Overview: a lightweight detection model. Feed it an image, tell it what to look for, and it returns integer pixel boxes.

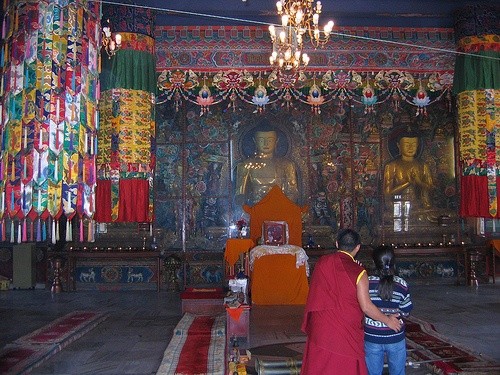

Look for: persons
[234,120,298,201]
[301,229,403,375]
[362,246,413,375]
[383,125,436,224]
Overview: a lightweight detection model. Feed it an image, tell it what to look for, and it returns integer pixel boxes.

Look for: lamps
[102,19,121,59]
[267,0,334,71]
